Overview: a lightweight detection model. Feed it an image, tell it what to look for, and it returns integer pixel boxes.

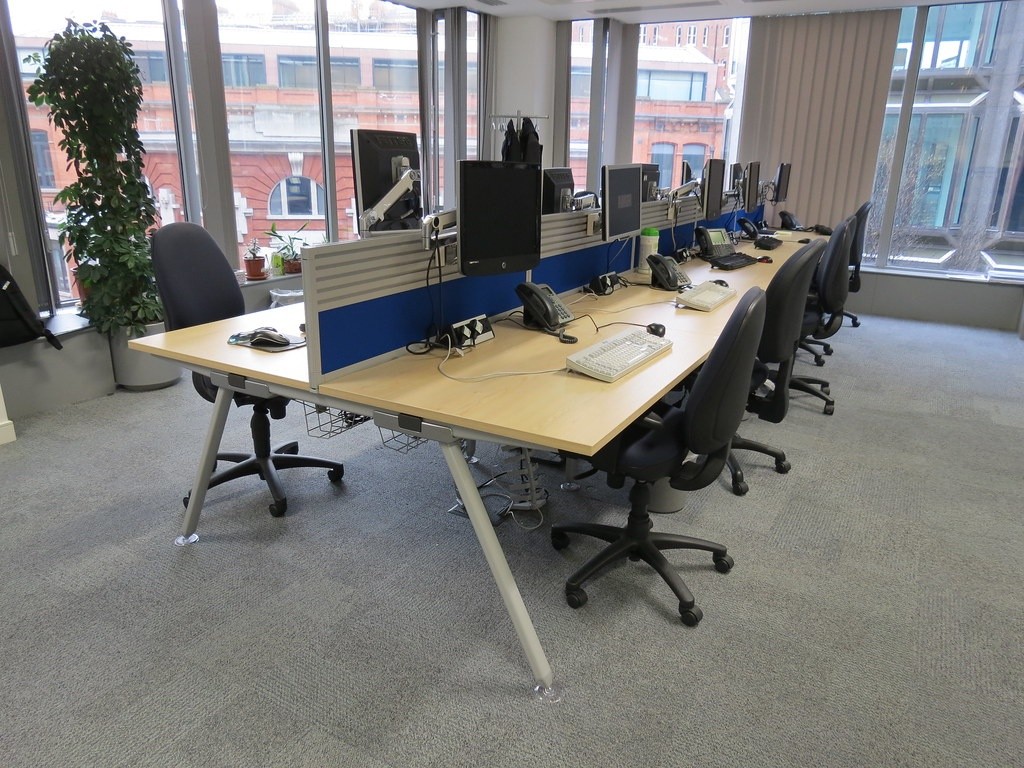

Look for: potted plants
[22,17,181,391]
[243,237,266,279]
[266,221,310,274]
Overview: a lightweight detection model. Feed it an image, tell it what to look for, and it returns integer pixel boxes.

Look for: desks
[127,191,835,704]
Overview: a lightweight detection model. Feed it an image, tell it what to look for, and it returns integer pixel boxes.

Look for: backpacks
[0,264,63,350]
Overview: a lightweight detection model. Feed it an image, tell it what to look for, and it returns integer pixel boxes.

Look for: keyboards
[710,252,758,270]
[675,281,736,312]
[754,236,783,250]
[566,327,673,383]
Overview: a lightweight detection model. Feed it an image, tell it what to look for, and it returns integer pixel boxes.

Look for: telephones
[694,226,735,257]
[645,253,693,290]
[779,210,800,228]
[515,282,575,330]
[736,217,759,238]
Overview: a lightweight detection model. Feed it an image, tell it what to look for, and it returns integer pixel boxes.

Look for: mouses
[250,329,290,346]
[646,323,665,337]
[713,279,728,287]
[798,239,812,243]
[757,255,773,263]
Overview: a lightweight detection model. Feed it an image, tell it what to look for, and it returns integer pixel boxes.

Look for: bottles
[639,228,660,274]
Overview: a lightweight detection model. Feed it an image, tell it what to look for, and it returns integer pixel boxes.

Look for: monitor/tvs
[681,160,691,196]
[541,167,575,215]
[773,163,792,202]
[703,159,725,221]
[350,129,423,236]
[642,163,660,202]
[601,163,641,241]
[729,162,743,191]
[742,161,760,213]
[455,159,541,276]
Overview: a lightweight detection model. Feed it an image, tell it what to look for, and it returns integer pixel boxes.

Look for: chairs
[757,214,858,415]
[843,201,874,329]
[548,286,766,628]
[667,237,829,496]
[149,221,345,522]
